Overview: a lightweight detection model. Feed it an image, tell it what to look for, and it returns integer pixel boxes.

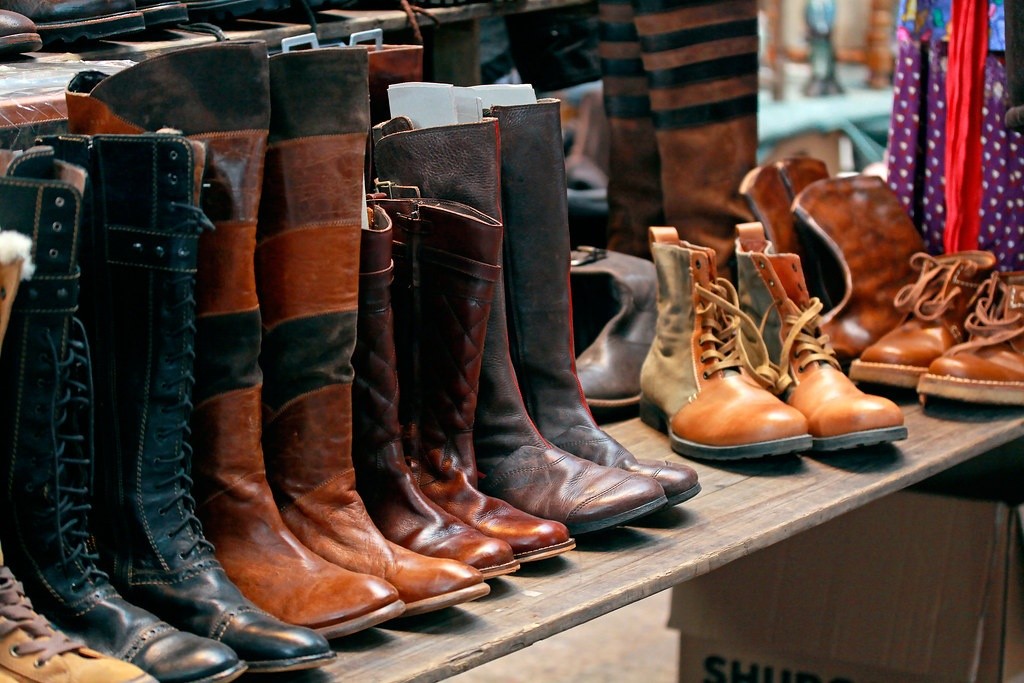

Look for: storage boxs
[666,489,1024,683]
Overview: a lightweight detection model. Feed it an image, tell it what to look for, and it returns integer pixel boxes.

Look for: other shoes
[0,0,290,55]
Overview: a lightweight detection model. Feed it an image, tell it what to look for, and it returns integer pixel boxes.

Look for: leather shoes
[849,250,999,389]
[916,270,1024,405]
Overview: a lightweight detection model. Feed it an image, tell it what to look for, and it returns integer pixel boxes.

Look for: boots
[0,14,701,683]
[571,0,930,455]
